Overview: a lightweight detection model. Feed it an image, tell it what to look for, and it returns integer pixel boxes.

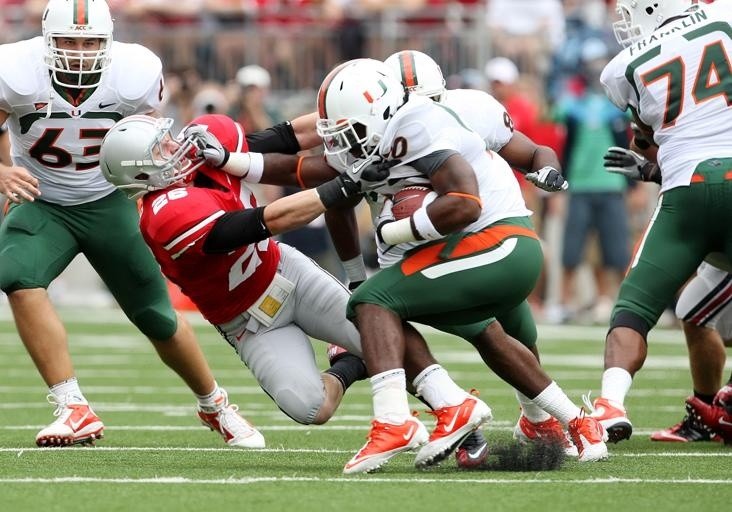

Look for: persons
[2,0,661,324]
[100,51,611,473]
[2,0,269,451]
[582,0,732,451]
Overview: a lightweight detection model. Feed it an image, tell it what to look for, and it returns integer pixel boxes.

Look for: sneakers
[416,393,491,469]
[344,416,429,474]
[199,406,268,448]
[514,412,578,460]
[687,388,729,443]
[35,405,104,447]
[456,431,488,467]
[567,415,608,463]
[652,408,721,442]
[592,399,634,443]
[535,301,613,324]
[327,342,372,380]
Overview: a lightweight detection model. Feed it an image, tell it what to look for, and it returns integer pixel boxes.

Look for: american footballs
[391,186,440,220]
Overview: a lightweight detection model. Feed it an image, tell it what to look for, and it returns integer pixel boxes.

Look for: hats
[486,57,521,83]
[238,66,271,87]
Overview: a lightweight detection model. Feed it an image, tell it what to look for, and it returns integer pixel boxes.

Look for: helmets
[314,56,404,180]
[611,2,689,48]
[99,115,199,197]
[40,0,116,91]
[385,50,447,99]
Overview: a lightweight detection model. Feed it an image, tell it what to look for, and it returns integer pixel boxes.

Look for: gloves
[374,200,395,238]
[525,166,569,193]
[339,156,401,199]
[603,146,656,181]
[190,126,227,169]
